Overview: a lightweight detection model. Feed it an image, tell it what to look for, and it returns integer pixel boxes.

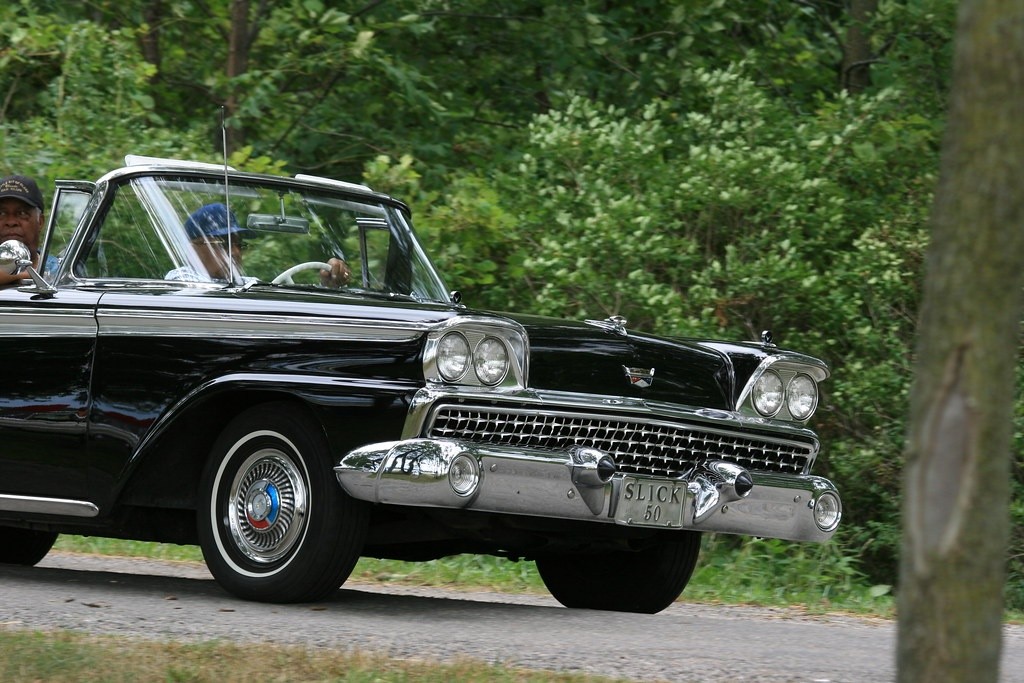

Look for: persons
[164,202,352,289]
[0,176,59,290]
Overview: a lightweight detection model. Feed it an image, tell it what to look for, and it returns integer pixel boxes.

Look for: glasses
[193,237,249,252]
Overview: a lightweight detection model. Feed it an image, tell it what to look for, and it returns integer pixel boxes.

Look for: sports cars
[0,154,844,614]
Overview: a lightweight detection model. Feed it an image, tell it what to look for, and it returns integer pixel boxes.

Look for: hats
[0,174,44,213]
[185,203,251,240]
